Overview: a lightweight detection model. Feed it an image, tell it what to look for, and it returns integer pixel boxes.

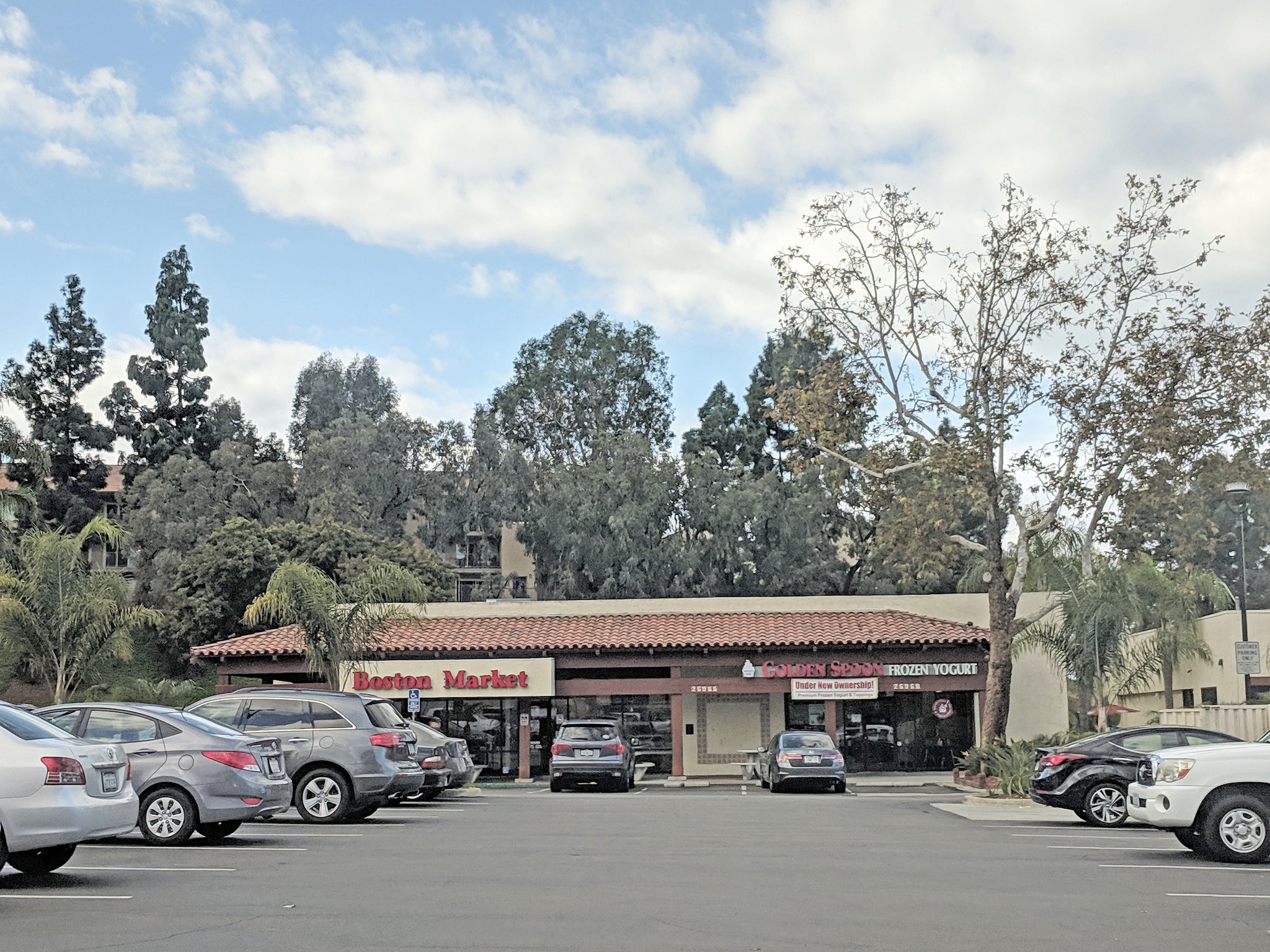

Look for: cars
[401,718,474,801]
[1030,725,1247,827]
[0,697,294,871]
[757,729,847,794]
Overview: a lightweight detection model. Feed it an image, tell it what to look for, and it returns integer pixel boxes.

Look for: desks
[730,748,760,795]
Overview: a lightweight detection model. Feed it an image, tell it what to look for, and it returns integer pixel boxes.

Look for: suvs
[1127,724,1269,865]
[426,707,501,744]
[549,719,635,792]
[171,686,425,824]
